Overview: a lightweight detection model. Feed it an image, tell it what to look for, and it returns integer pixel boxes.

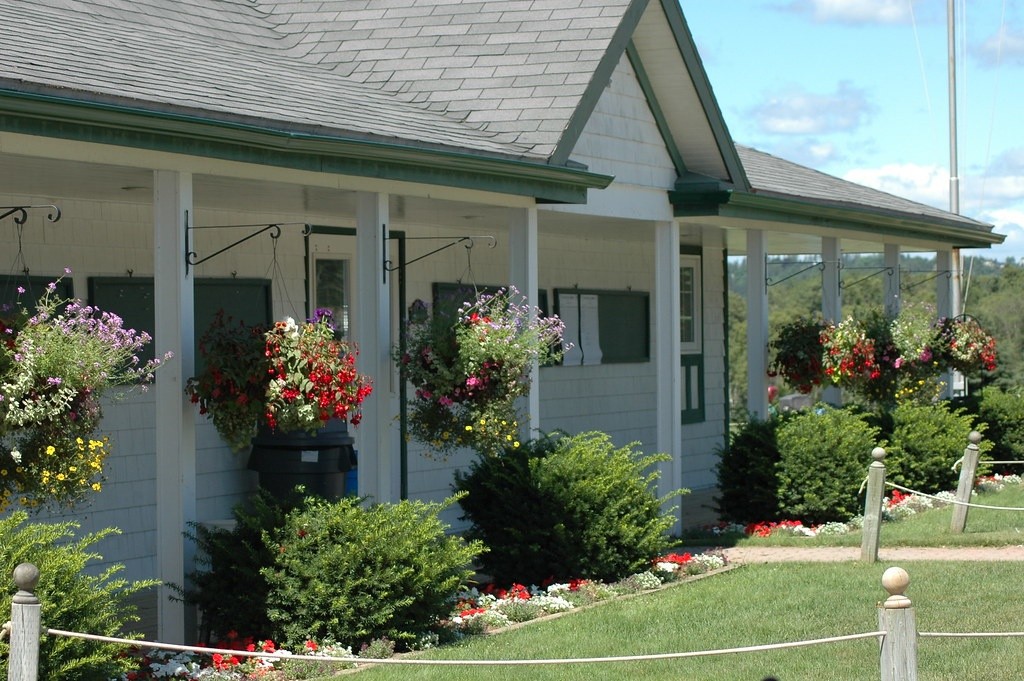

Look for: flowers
[763,294,998,407]
[389,285,576,466]
[181,306,372,456]
[0,265,178,524]
